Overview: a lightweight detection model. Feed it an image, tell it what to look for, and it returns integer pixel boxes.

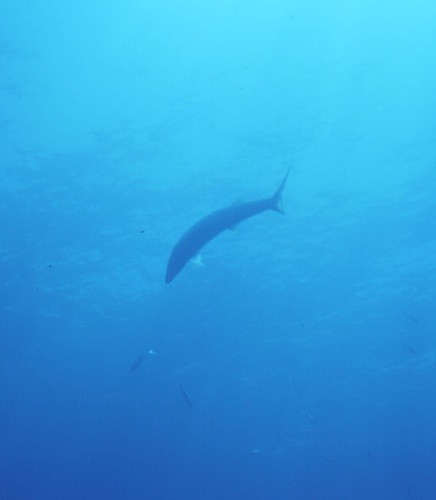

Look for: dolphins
[178,384,194,410]
[165,166,291,286]
[129,350,183,373]
